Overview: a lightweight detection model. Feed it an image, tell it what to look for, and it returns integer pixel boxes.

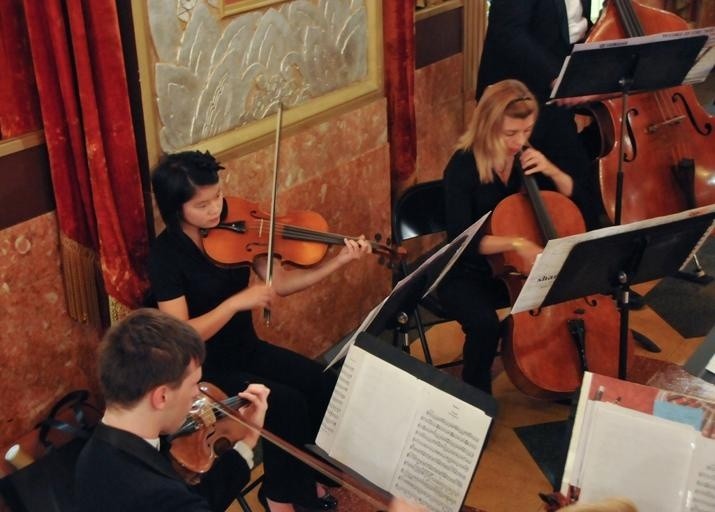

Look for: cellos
[487,149,635,399]
[582,1,715,225]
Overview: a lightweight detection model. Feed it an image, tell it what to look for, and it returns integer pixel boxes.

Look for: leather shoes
[318,489,339,511]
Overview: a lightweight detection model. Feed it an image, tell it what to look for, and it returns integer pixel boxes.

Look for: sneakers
[628,288,646,310]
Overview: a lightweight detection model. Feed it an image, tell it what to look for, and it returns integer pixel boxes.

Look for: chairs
[136,289,265,512]
[392,180,504,371]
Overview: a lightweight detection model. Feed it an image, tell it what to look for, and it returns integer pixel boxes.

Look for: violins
[168,381,249,485]
[201,196,408,269]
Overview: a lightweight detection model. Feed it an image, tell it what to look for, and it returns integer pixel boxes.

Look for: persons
[72,309,270,512]
[147,152,371,512]
[476,0,643,310]
[442,80,587,397]
